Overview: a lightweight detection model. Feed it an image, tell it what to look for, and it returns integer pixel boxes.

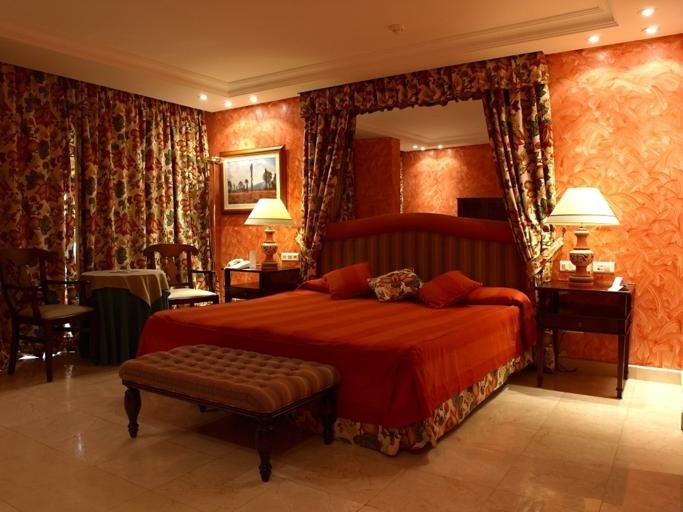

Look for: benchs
[119,344,341,482]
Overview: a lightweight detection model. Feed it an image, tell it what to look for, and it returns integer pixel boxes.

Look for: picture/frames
[219,145,286,215]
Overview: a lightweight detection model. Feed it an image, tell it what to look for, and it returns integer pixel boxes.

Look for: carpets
[204,412,316,458]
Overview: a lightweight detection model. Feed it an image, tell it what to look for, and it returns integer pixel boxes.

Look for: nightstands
[534,280,636,398]
[221,266,300,303]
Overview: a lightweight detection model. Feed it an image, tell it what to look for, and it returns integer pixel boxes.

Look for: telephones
[223,259,251,270]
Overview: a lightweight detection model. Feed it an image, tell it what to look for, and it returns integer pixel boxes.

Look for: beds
[135,212,537,457]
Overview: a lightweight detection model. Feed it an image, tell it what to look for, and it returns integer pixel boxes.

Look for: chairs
[0,246,96,382]
[144,244,219,308]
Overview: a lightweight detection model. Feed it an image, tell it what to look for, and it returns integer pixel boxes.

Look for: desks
[80,269,170,365]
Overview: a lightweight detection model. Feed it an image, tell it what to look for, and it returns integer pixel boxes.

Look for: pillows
[302,262,529,305]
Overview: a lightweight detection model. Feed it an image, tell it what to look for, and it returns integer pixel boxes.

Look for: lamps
[545,187,621,287]
[244,198,293,266]
